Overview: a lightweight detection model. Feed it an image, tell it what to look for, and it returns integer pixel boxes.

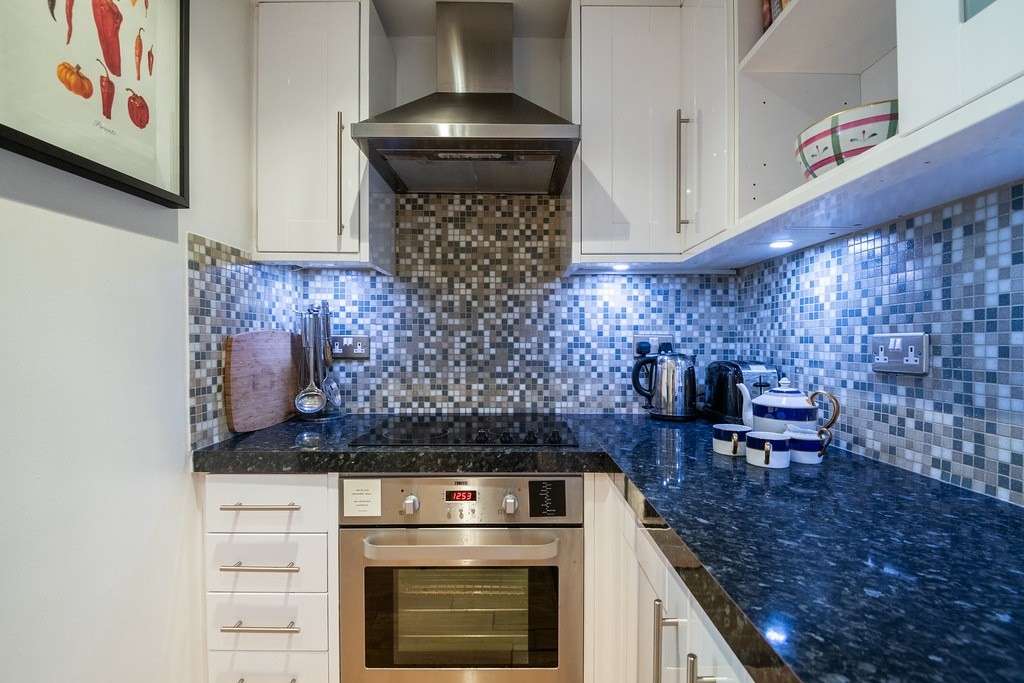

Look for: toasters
[703,361,779,424]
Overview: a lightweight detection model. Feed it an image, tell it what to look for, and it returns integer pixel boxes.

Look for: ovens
[338,476,583,683]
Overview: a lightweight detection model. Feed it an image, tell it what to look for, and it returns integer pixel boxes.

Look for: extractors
[351,91,581,194]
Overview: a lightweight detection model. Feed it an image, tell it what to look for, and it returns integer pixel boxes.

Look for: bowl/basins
[795,99,899,180]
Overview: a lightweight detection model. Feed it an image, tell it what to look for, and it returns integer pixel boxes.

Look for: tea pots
[736,375,840,433]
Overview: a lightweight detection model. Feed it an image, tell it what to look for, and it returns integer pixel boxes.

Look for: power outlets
[331,335,370,360]
[870,332,931,375]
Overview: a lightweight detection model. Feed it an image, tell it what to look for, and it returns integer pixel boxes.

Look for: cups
[783,428,832,464]
[713,425,752,456]
[746,432,790,469]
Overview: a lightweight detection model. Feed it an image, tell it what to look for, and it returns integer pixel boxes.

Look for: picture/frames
[1,0,190,211]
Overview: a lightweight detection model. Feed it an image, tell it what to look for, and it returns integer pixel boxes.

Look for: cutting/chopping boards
[224,329,302,433]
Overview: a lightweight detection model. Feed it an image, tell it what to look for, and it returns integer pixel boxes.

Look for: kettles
[632,353,696,422]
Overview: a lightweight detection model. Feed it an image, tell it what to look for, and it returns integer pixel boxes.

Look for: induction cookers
[348,413,580,447]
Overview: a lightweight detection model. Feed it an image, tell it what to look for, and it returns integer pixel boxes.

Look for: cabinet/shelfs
[682,0,770,276]
[205,471,340,683]
[623,505,742,683]
[252,0,398,277]
[733,0,1024,261]
[560,0,684,277]
